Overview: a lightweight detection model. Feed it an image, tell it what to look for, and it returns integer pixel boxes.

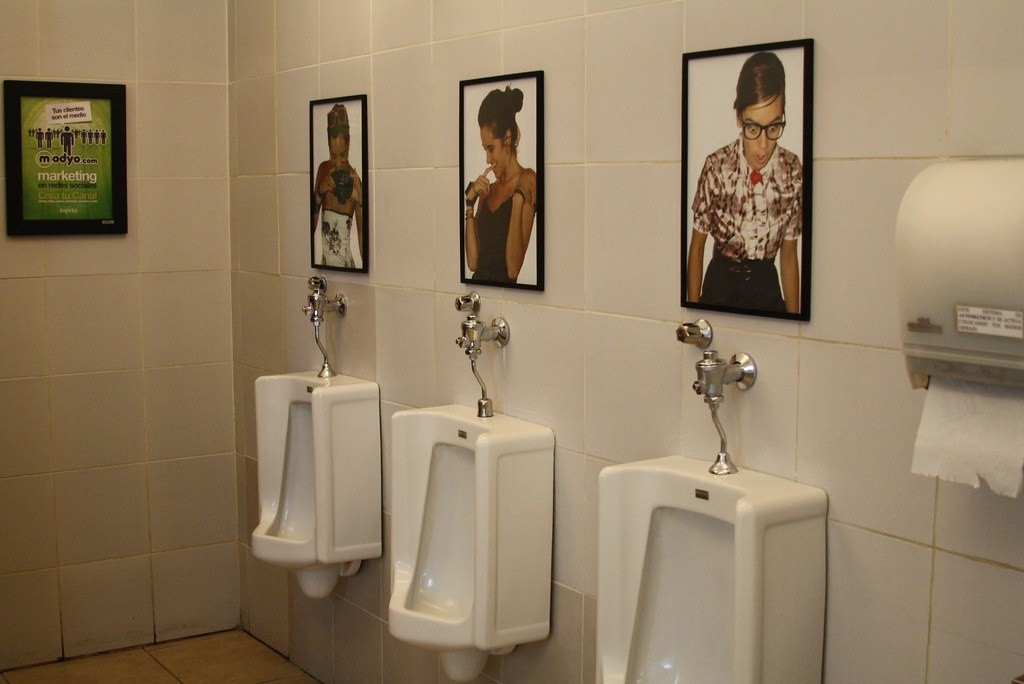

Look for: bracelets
[465,199,474,219]
[514,189,525,203]
[317,184,328,200]
[473,185,479,195]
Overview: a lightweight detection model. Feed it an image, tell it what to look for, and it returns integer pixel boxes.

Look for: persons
[314,104,363,269]
[465,85,536,283]
[687,52,803,313]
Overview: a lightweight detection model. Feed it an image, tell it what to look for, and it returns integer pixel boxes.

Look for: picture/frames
[309,93,369,274]
[458,71,545,291]
[4,79,127,235]
[680,38,813,324]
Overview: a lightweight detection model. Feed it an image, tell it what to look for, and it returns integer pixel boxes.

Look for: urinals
[593,453,829,684]
[250,373,383,600]
[390,405,551,681]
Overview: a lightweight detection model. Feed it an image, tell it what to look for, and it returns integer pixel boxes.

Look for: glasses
[327,108,347,128]
[741,109,786,140]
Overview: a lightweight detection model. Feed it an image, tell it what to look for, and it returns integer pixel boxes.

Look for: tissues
[891,157,1023,500]
